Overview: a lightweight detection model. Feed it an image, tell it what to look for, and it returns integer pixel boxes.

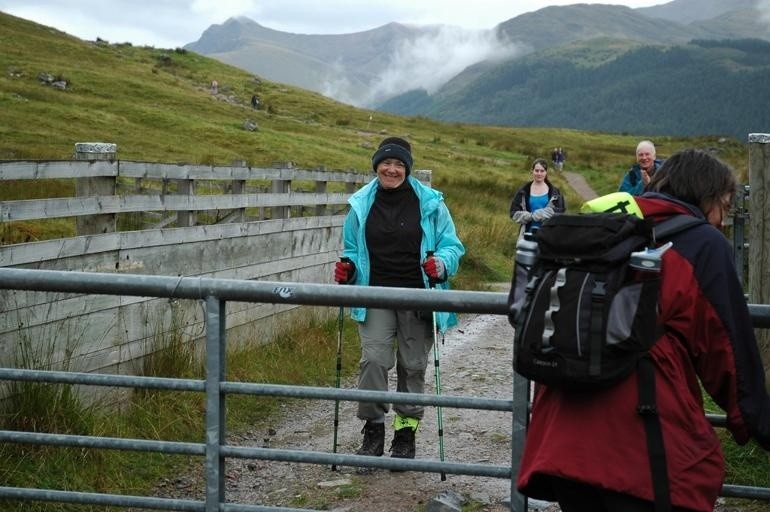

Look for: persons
[618,139,665,196]
[256,97,261,110]
[211,80,218,94]
[252,96,256,109]
[509,159,565,247]
[551,148,559,170]
[558,147,564,171]
[334,137,467,473]
[516,149,770,512]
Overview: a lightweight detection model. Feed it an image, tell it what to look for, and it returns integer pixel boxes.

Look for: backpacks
[513,211,708,390]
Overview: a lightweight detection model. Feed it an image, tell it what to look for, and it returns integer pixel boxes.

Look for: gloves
[335,262,353,283]
[513,207,554,224]
[422,257,444,279]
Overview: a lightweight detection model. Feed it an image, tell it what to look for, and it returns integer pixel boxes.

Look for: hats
[372,137,413,176]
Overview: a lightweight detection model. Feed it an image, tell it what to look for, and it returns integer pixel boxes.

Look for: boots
[358,421,384,456]
[390,414,419,471]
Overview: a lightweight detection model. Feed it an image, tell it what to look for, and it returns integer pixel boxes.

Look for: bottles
[512,226,541,303]
[630,248,665,281]
